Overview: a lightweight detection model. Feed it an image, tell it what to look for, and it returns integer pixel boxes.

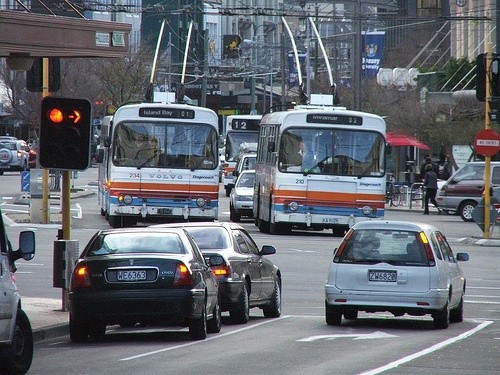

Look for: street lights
[243,39,274,113]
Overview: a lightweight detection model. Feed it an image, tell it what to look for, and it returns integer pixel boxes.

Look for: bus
[252,15,391,236]
[223,113,262,196]
[96,15,219,227]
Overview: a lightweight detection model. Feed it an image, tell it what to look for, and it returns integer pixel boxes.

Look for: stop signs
[472,128,500,158]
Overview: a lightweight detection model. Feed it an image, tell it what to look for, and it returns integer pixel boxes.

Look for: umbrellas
[385,129,430,181]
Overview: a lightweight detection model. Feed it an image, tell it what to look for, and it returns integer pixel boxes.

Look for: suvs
[231,142,258,184]
[0,135,38,175]
[434,161,500,221]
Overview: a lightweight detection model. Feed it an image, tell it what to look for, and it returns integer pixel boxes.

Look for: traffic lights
[39,96,91,171]
[222,35,242,59]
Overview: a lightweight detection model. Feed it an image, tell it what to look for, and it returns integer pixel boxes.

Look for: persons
[315,132,337,164]
[421,146,449,215]
[296,141,313,166]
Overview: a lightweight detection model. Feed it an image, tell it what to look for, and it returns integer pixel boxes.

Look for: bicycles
[385,173,402,207]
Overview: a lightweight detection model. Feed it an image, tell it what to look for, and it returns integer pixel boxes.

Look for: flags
[361,31,385,79]
[286,51,306,91]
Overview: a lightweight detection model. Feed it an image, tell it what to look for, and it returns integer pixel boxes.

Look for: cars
[66,221,284,344]
[229,170,255,222]
[325,219,470,329]
[0,209,36,375]
